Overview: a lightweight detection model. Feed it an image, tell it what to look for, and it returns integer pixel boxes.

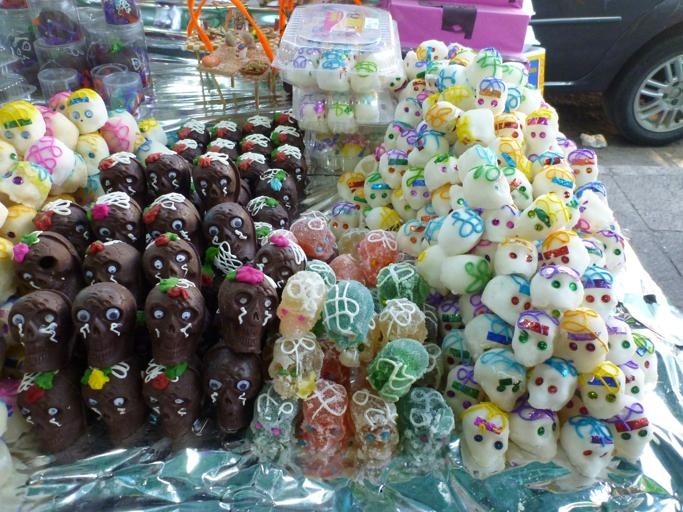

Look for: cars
[521,0,683,151]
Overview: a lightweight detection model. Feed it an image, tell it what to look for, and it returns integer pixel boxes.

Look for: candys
[0,35,659,480]
